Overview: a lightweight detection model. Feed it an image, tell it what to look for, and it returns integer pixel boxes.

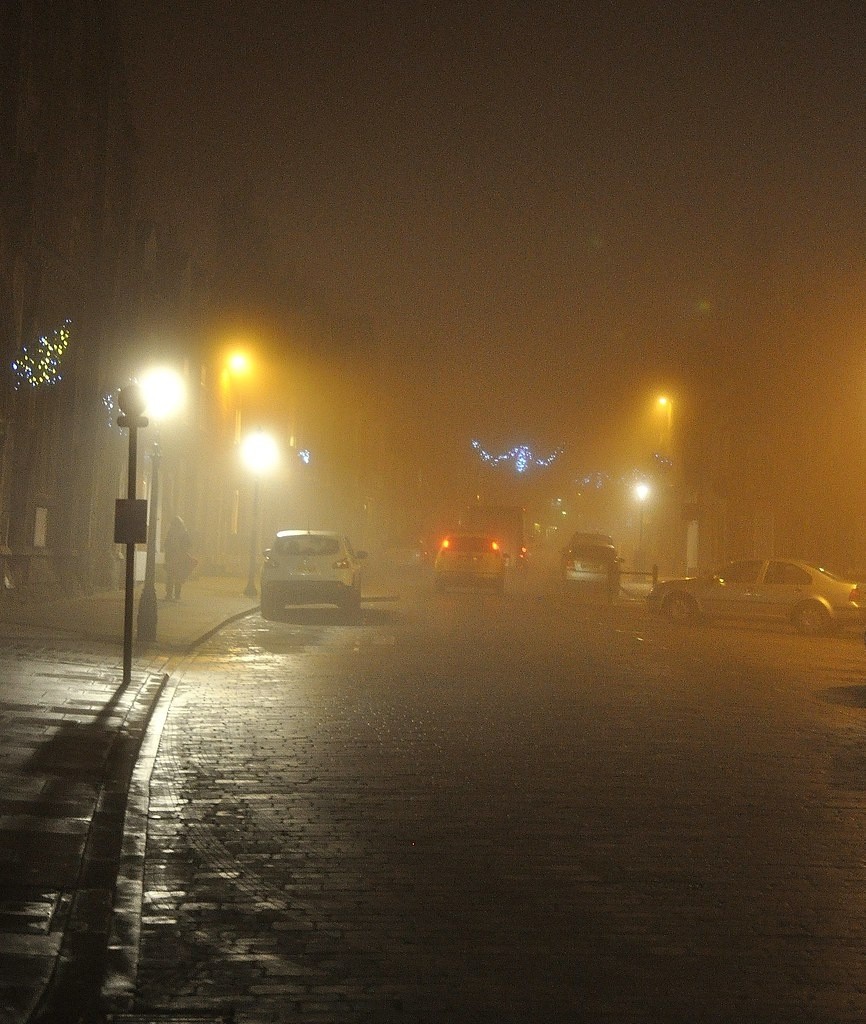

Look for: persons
[162,516,188,601]
[184,511,204,582]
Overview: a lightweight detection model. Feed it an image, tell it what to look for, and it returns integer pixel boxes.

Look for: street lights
[238,432,279,595]
[633,484,649,547]
[135,362,186,643]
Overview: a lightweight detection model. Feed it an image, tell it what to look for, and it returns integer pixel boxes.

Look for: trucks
[454,502,534,576]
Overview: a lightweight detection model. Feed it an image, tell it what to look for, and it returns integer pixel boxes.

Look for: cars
[541,530,626,595]
[260,530,368,619]
[646,553,864,636]
[434,530,510,595]
